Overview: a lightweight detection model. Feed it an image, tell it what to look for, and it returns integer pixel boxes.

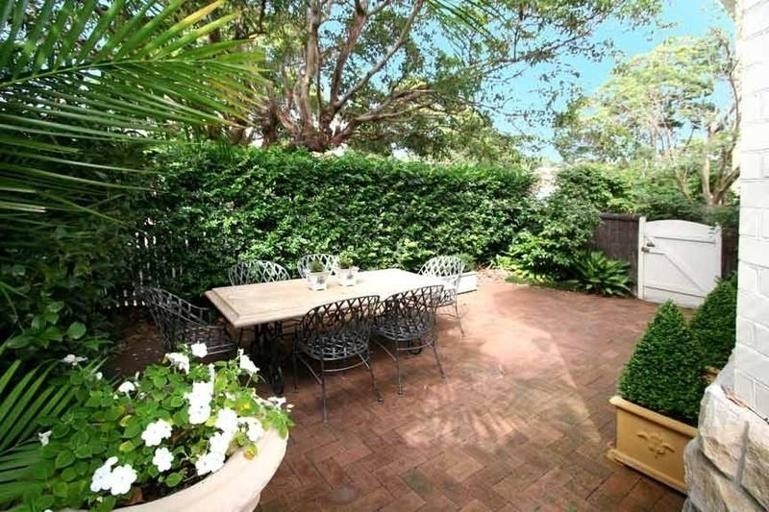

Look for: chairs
[372,285,448,394]
[297,253,348,279]
[295,296,384,427]
[136,285,250,386]
[228,260,291,339]
[415,255,465,339]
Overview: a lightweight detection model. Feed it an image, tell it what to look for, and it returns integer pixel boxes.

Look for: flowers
[44,339,295,496]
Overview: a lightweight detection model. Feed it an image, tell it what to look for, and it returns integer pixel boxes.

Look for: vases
[101,426,289,512]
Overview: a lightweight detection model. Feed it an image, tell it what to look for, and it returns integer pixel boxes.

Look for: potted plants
[688,280,737,386]
[304,260,331,291]
[609,300,701,493]
[335,252,361,288]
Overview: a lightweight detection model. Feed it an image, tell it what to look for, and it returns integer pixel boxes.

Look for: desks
[205,268,445,394]
